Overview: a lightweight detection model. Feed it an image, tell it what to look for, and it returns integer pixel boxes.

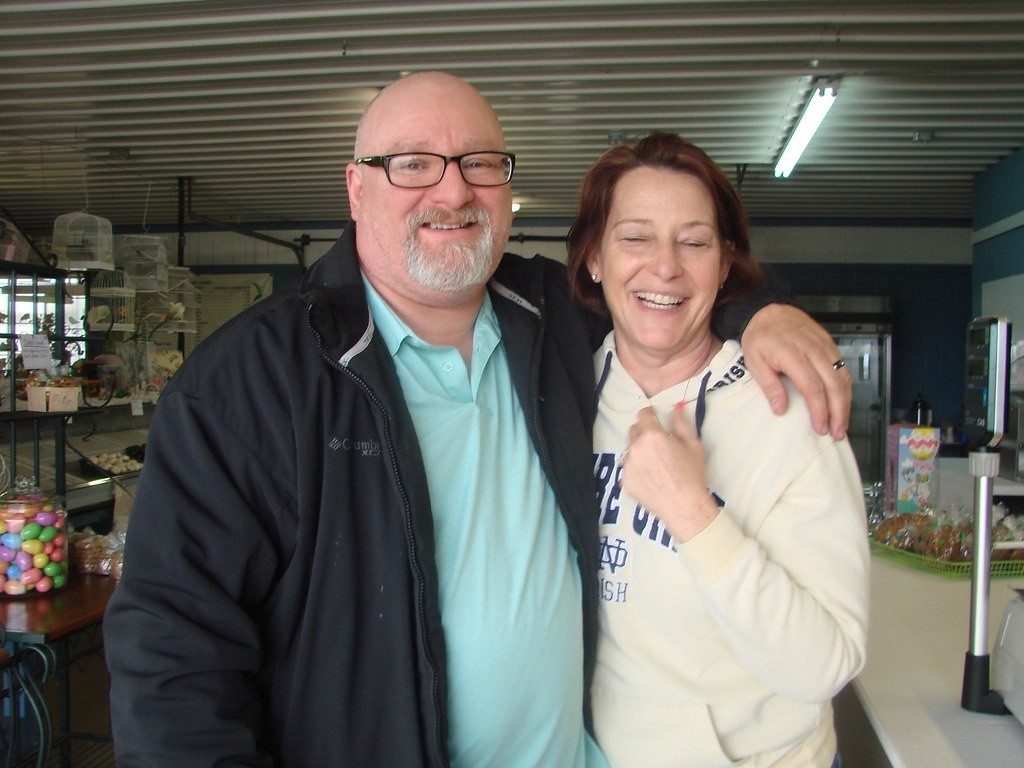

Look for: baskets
[64,525,96,564]
[73,532,109,575]
[870,536,1024,581]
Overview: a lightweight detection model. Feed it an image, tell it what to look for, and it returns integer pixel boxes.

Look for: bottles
[0,475,69,598]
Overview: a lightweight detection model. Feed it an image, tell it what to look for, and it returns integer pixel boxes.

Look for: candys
[0,495,67,593]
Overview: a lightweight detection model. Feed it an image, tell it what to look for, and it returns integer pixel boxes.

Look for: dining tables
[840,482,1024,768]
[0,528,120,768]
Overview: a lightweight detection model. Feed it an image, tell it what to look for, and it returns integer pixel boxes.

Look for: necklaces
[641,369,691,437]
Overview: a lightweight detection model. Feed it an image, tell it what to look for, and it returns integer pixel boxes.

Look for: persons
[102,71,852,768]
[564,128,870,768]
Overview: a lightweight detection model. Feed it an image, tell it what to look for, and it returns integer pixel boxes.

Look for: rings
[832,359,845,370]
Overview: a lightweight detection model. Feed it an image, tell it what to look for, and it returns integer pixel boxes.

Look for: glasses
[352,151,518,189]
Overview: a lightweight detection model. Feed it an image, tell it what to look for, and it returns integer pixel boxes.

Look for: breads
[27,374,76,403]
[876,514,1023,563]
